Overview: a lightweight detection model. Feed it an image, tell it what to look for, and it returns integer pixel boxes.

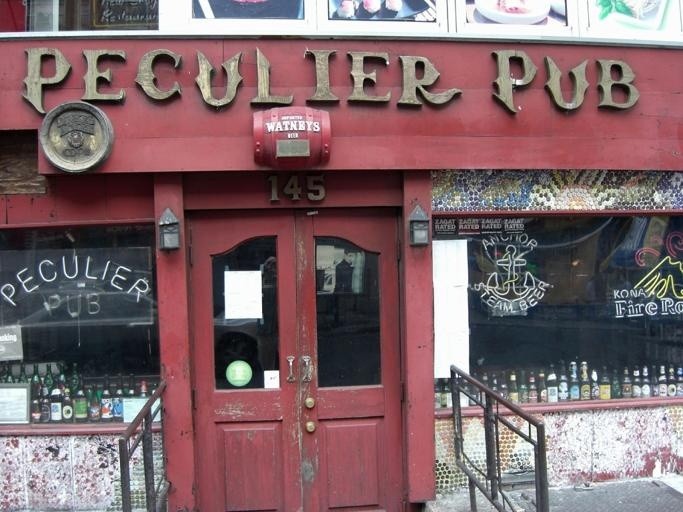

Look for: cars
[7,268,262,378]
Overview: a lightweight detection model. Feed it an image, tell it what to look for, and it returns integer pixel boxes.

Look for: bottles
[0,356,161,424]
[433,357,683,412]
